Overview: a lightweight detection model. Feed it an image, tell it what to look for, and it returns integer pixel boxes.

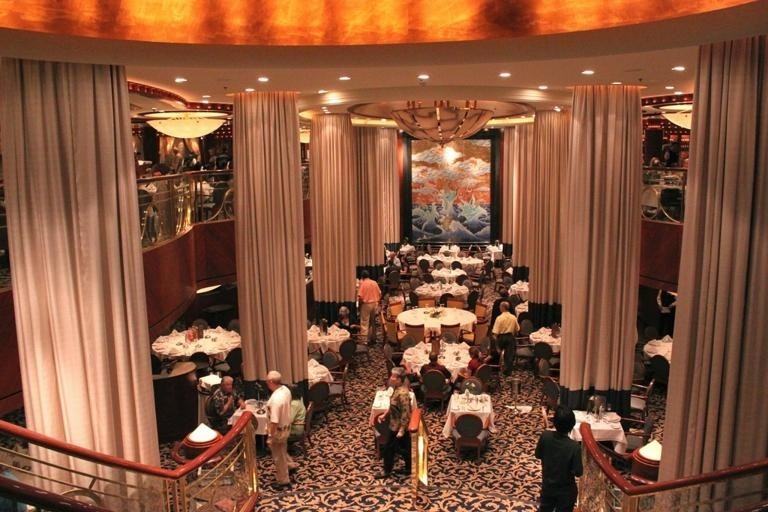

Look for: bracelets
[398,430,405,432]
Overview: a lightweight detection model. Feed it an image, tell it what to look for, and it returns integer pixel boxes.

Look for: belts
[277,427,289,432]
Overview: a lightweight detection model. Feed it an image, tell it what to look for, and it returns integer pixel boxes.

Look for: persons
[374,366,412,479]
[491,289,515,328]
[491,301,520,377]
[205,376,245,435]
[482,336,499,372]
[420,351,451,380]
[334,306,361,339]
[356,269,382,345]
[652,147,678,167]
[385,260,400,284]
[494,268,509,297]
[464,347,482,377]
[266,370,299,489]
[535,405,584,512]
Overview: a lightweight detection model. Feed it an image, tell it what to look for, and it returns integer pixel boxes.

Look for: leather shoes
[271,483,292,491]
[289,466,300,477]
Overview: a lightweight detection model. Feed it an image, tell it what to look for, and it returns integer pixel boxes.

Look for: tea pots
[373,401,382,408]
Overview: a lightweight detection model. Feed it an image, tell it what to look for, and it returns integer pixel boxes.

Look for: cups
[407,305,411,312]
[454,391,459,400]
[389,382,395,390]
[440,304,444,308]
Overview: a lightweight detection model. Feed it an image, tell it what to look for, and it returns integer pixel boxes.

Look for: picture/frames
[403,129,501,244]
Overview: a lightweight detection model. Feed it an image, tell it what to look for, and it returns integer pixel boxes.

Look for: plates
[468,404,480,410]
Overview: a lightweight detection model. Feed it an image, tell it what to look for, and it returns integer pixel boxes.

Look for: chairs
[405,323,432,345]
[418,259,430,272]
[460,376,483,395]
[383,343,403,378]
[535,354,560,382]
[228,319,241,333]
[190,352,209,378]
[621,411,657,451]
[518,311,532,335]
[189,351,213,377]
[384,319,405,345]
[350,323,374,366]
[339,339,357,374]
[433,324,460,346]
[399,372,421,402]
[646,355,670,391]
[378,307,398,348]
[471,271,486,287]
[422,370,452,413]
[388,301,404,320]
[417,296,438,307]
[328,363,349,411]
[474,363,491,380]
[461,319,489,347]
[397,333,418,351]
[487,349,506,385]
[432,260,445,269]
[286,402,315,455]
[195,180,229,221]
[307,382,330,426]
[534,342,560,367]
[374,414,391,460]
[539,376,560,404]
[455,414,488,462]
[451,260,462,270]
[151,354,178,374]
[629,379,656,428]
[191,319,208,329]
[386,267,400,295]
[438,331,458,343]
[204,395,231,433]
[492,263,512,282]
[474,301,488,321]
[307,344,322,361]
[541,406,555,432]
[318,350,341,378]
[493,271,512,292]
[514,337,534,369]
[446,297,466,310]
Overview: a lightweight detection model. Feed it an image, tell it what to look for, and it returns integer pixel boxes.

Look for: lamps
[408,410,429,489]
[657,104,693,130]
[182,422,223,450]
[632,440,662,467]
[378,98,496,148]
[140,112,230,139]
